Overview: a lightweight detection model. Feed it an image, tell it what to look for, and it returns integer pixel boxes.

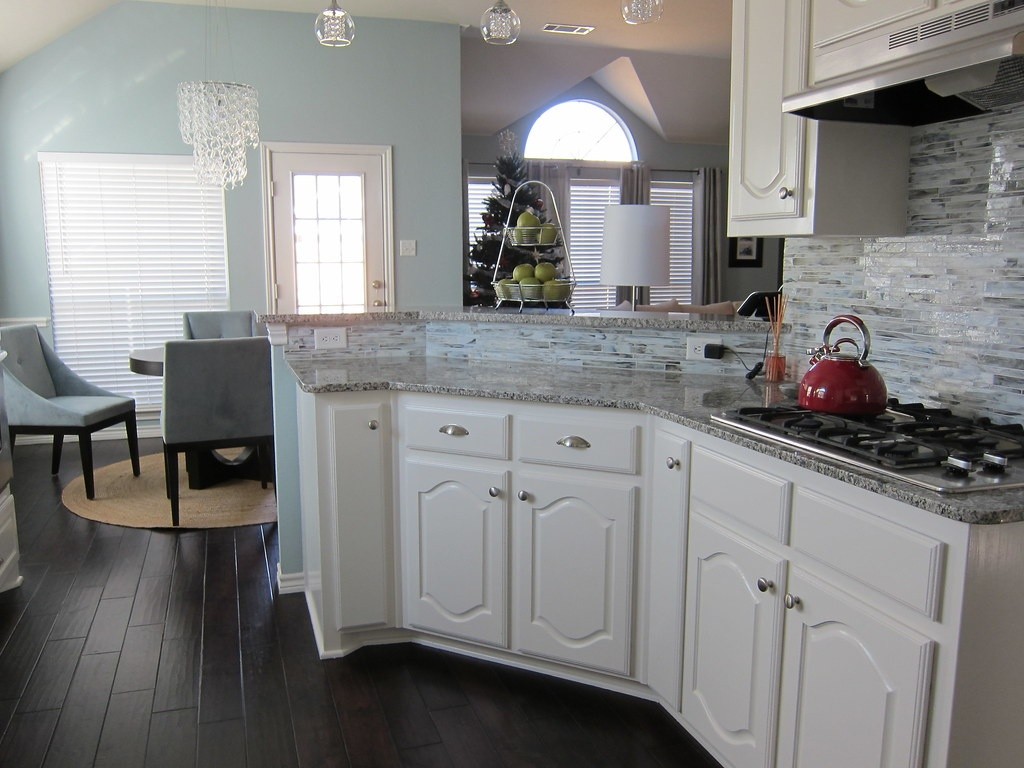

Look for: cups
[765,355,786,382]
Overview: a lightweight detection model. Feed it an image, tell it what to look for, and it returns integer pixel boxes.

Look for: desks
[130,348,272,490]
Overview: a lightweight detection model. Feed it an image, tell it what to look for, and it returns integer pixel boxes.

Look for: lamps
[620,0,665,25]
[481,0,521,45]
[315,0,355,47]
[599,205,670,310]
[174,0,260,191]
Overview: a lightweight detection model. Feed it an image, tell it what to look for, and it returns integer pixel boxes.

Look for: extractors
[781,0,1024,128]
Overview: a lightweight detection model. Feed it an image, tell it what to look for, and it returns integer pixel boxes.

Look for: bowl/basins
[779,383,799,399]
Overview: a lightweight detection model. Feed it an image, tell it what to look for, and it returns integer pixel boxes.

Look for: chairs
[158,337,274,527]
[0,324,141,499]
[183,309,255,340]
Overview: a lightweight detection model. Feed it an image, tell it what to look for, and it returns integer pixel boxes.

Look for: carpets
[61,452,277,528]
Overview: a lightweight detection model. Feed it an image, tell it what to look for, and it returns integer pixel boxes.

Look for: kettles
[798,314,887,414]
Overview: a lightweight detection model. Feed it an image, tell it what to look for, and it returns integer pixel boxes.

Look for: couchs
[612,301,744,314]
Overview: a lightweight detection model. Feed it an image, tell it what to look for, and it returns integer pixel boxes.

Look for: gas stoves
[710,398,1024,492]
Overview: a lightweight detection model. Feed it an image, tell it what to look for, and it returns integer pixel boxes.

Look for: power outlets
[685,336,725,360]
[314,328,347,350]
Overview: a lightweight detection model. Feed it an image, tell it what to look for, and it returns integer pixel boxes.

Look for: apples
[513,211,558,244]
[496,261,569,301]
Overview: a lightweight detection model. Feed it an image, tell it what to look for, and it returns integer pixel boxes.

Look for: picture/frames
[728,235,764,270]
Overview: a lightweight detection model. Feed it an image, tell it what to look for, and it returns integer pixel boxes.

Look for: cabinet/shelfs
[726,1,908,240]
[293,383,1024,767]
